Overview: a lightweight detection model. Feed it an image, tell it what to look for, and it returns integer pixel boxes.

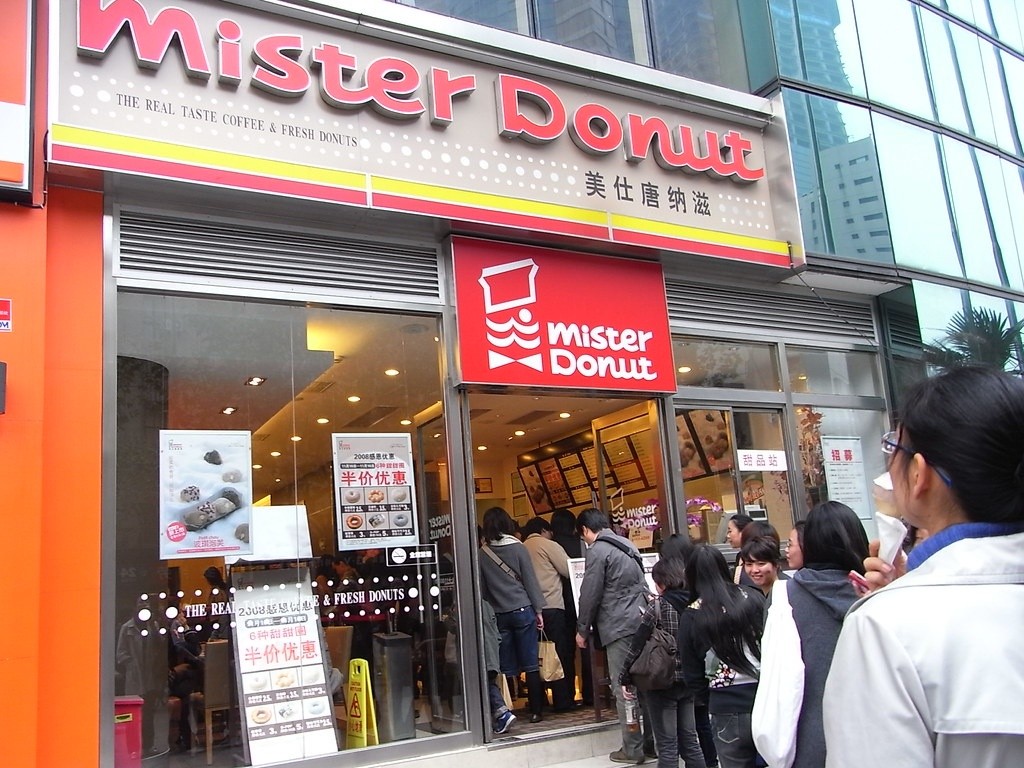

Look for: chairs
[188,638,238,766]
[324,626,353,715]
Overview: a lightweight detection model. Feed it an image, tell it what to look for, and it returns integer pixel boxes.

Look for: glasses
[880,431,952,487]
[786,541,799,548]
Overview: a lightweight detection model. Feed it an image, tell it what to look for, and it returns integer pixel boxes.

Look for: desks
[177,641,204,672]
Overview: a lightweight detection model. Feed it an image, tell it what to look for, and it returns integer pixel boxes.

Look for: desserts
[180,449,250,543]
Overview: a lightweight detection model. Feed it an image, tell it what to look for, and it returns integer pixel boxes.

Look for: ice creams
[873,471,903,521]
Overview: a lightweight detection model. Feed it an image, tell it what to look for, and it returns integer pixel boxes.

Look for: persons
[819,365,1024,768]
[576,500,931,768]
[314,506,583,735]
[116,567,235,761]
[117,592,167,759]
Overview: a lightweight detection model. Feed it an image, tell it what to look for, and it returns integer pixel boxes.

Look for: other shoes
[529,713,542,722]
[644,748,657,757]
[494,711,517,734]
[610,747,645,764]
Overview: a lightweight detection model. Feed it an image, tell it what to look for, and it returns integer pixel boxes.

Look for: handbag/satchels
[628,595,678,690]
[496,670,514,711]
[444,631,457,663]
[751,579,805,768]
[536,629,565,682]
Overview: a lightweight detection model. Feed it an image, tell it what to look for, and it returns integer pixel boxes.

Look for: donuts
[345,489,408,529]
[247,668,325,724]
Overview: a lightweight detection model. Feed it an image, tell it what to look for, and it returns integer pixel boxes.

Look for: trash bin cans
[370,630,417,743]
